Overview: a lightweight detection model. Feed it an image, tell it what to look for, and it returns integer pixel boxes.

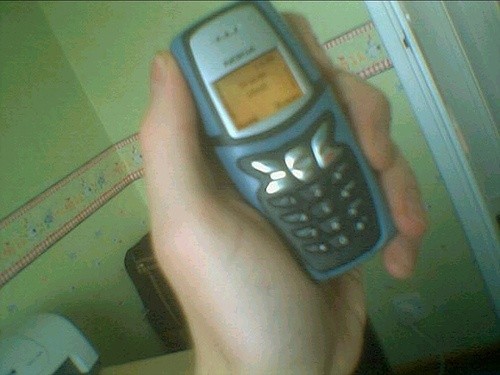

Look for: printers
[0,313,102,375]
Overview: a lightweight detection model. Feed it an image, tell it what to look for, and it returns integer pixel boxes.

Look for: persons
[140,0,427,375]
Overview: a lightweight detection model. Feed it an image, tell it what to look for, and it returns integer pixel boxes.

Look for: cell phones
[170,0,399,283]
